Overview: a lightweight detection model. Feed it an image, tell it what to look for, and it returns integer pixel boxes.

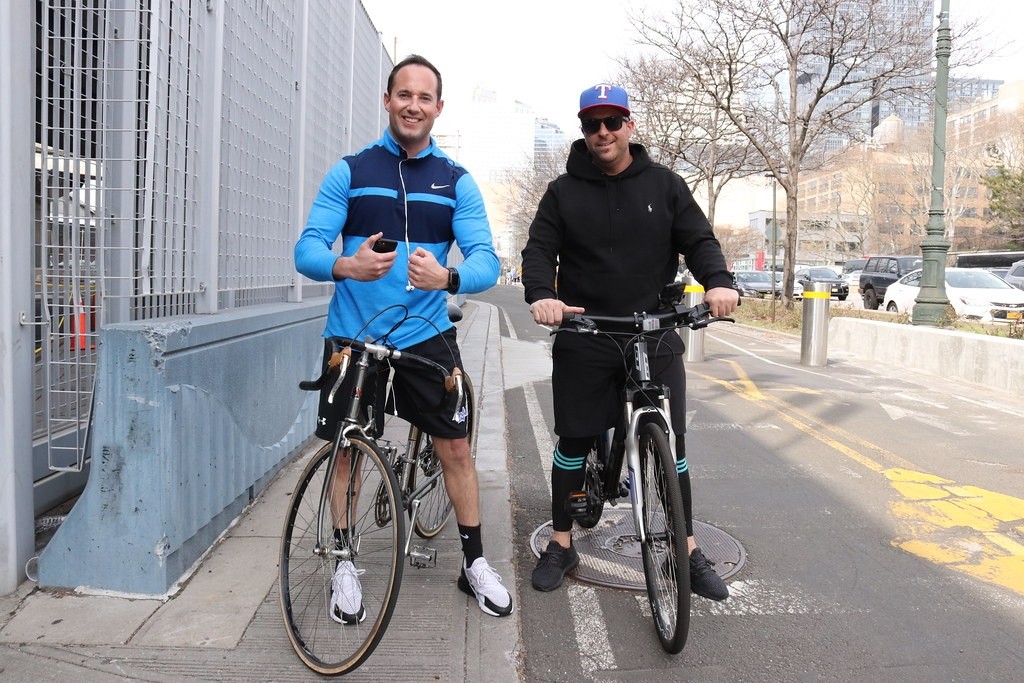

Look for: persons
[521,83,741,601]
[294,55,513,625]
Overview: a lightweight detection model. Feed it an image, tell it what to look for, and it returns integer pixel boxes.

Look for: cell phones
[373,239,398,253]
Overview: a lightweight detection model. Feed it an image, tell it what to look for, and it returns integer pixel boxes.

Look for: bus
[956,252,1024,277]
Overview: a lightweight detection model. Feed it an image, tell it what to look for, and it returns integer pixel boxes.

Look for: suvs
[842,259,894,286]
[858,255,924,310]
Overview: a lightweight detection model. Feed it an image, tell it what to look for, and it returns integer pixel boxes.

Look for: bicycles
[277,305,473,678]
[549,289,741,655]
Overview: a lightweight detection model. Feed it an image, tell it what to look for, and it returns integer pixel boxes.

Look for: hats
[577,83,630,119]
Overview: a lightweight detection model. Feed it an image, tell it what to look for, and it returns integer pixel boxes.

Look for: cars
[1003,259,1024,290]
[731,265,849,301]
[884,267,1024,322]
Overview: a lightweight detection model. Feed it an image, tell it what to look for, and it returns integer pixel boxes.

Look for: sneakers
[330,561,366,625]
[661,547,729,601]
[457,555,514,616]
[531,534,580,592]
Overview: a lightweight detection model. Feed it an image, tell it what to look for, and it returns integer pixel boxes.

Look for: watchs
[448,266,459,295]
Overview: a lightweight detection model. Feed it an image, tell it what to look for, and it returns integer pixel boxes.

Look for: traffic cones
[70,292,95,350]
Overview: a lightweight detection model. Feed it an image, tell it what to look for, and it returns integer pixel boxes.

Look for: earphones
[406,285,414,291]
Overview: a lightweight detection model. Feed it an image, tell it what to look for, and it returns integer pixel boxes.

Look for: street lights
[764,170,789,323]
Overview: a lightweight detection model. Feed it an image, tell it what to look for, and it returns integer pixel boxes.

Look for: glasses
[581,115,629,134]
